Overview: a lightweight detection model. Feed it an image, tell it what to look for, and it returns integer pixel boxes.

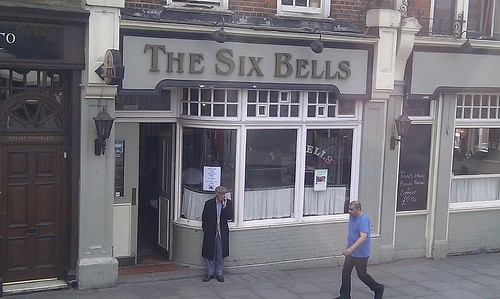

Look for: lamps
[390,113,411,150]
[455,31,472,48]
[212,15,227,43]
[93,106,115,155]
[306,27,324,53]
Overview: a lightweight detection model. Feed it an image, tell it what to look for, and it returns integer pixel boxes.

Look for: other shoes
[375,284,384,299]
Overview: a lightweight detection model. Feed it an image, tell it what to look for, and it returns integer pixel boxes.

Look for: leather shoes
[203,274,214,281]
[215,275,224,281]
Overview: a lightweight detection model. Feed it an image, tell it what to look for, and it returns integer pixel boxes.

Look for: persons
[334,201,384,299]
[202,186,233,282]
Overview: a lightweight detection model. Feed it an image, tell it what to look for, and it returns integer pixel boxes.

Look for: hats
[215,186,227,193]
